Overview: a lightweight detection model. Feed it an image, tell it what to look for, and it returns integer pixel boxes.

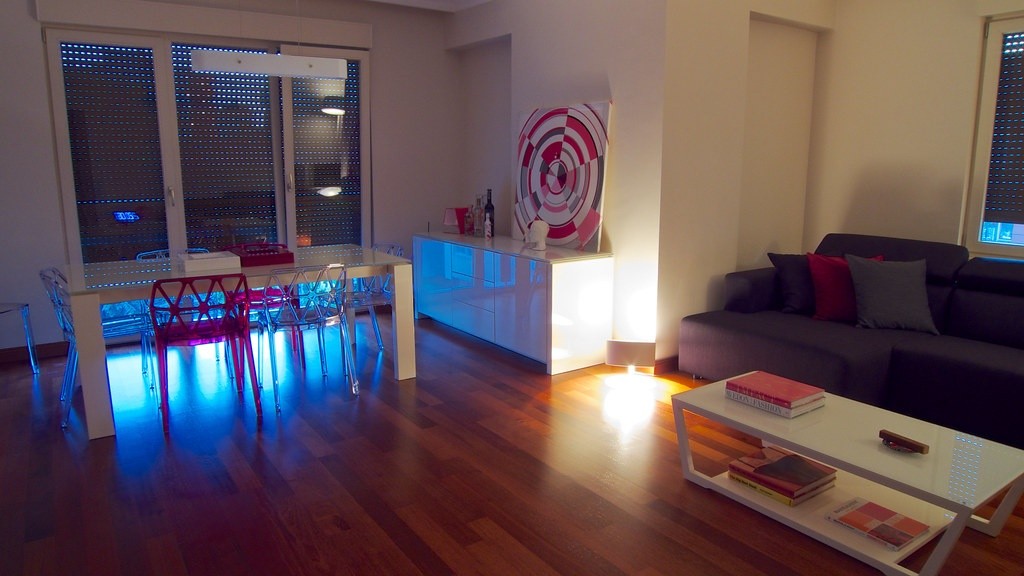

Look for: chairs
[147,273,263,440]
[255,262,362,413]
[333,240,402,352]
[40,267,159,431]
[226,242,306,372]
[136,246,238,412]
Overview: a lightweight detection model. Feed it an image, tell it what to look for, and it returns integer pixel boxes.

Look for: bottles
[464,204,474,236]
[473,198,483,237]
[484,189,495,238]
[484,213,491,241]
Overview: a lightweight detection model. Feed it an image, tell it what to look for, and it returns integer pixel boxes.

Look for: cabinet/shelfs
[412,236,614,375]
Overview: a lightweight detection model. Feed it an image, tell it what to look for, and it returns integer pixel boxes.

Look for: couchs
[677,233,1024,450]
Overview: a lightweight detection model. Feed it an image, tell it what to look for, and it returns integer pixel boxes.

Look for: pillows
[843,252,931,335]
[767,253,815,315]
[806,252,885,323]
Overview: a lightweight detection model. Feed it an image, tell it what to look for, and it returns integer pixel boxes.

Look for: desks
[670,370,1024,576]
[65,242,416,441]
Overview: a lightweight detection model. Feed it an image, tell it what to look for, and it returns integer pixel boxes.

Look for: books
[824,496,928,551]
[728,444,838,497]
[728,471,838,506]
[725,370,825,419]
